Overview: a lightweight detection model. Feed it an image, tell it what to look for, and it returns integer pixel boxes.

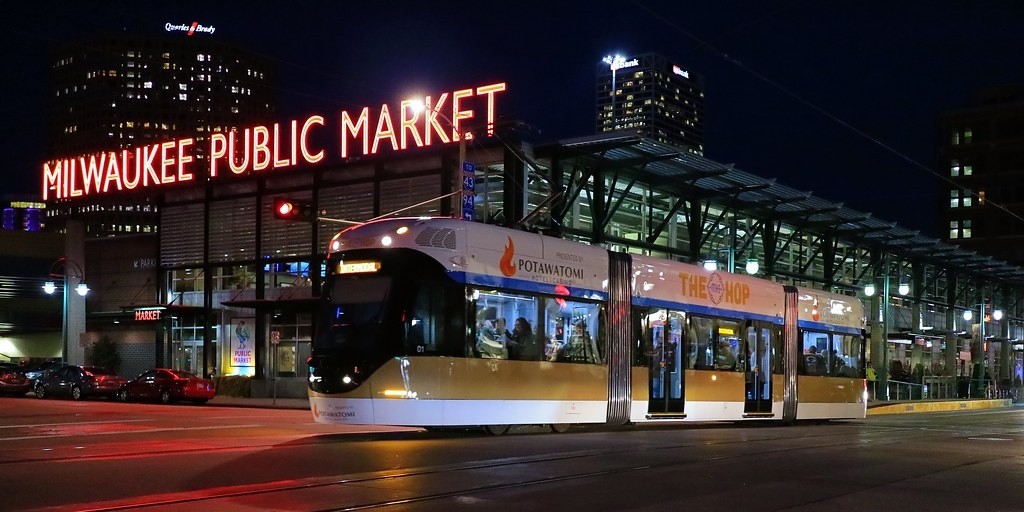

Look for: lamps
[745,251,760,275]
[963,307,972,320]
[864,279,874,296]
[41,277,58,294]
[703,253,718,272]
[898,279,910,296]
[993,306,1003,321]
[75,280,91,296]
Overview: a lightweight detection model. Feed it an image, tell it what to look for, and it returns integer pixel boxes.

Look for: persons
[865,361,877,401]
[652,330,846,399]
[985,371,1022,400]
[475,318,593,363]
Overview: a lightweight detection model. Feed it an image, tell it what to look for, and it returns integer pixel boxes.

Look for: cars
[113,367,216,407]
[0,361,59,396]
[33,364,126,402]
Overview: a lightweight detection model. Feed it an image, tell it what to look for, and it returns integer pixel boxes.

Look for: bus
[307,217,874,442]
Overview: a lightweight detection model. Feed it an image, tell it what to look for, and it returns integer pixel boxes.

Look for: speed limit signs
[460,161,475,219]
